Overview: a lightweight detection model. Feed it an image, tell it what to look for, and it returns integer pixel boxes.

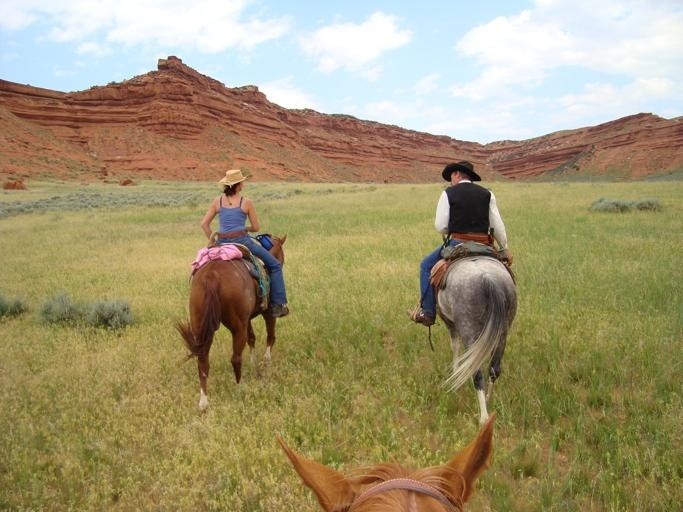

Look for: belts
[219,231,247,238]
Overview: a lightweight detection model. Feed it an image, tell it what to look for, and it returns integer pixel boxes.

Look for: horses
[175,233,289,414]
[274,409,498,512]
[433,233,517,424]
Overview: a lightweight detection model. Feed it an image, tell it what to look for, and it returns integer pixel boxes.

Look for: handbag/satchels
[255,234,273,250]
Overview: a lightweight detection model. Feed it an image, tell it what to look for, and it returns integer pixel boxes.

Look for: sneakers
[269,303,288,317]
[442,160,482,181]
[415,311,434,324]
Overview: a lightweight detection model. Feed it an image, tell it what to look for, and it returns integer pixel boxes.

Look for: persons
[411,159,513,325]
[201,170,289,318]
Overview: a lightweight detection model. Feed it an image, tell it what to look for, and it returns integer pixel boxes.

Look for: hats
[220,170,248,186]
